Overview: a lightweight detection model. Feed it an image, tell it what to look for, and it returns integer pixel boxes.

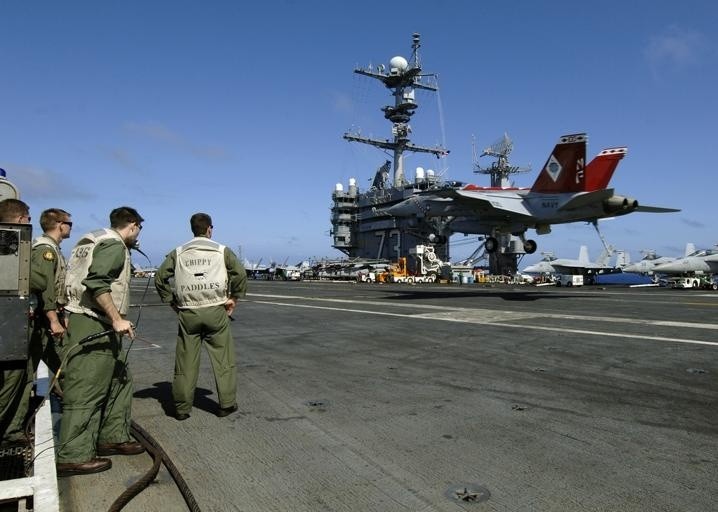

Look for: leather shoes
[96,440,146,456]
[56,457,112,477]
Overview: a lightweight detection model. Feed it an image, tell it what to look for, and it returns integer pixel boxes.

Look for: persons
[155,213,248,420]
[0,198,36,445]
[55,205,144,478]
[31,207,73,394]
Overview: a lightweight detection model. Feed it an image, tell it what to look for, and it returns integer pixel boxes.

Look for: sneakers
[174,411,190,421]
[218,402,238,417]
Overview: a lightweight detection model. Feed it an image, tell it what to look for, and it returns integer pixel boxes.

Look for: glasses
[24,216,31,222]
[55,220,73,227]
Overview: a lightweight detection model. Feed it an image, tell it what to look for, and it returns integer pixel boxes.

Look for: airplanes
[521,242,718,291]
[244,252,392,280]
[383,131,682,257]
[132,262,159,277]
